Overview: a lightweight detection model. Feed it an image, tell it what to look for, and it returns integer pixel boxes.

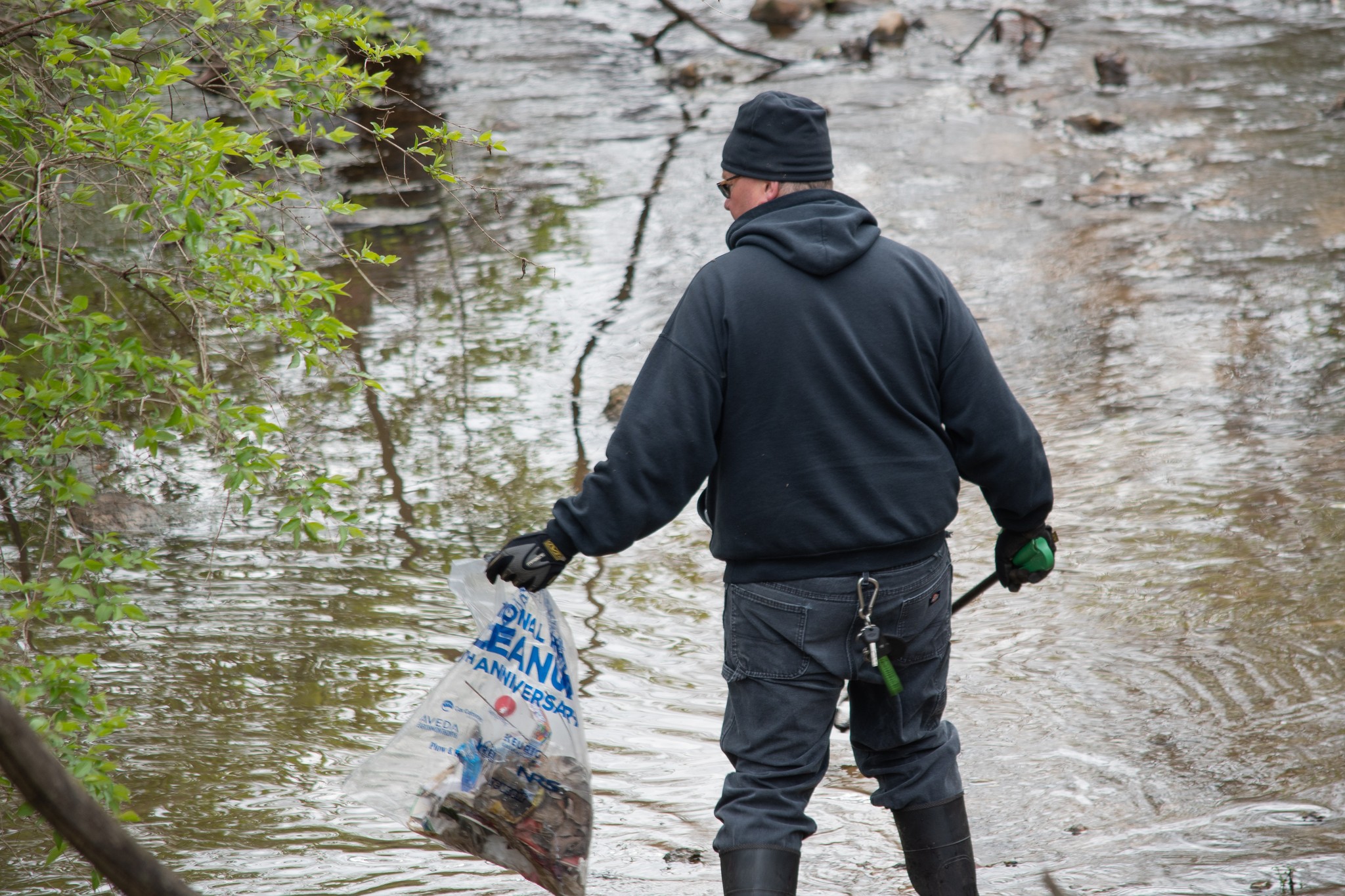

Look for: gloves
[484,529,572,592]
[995,521,1056,593]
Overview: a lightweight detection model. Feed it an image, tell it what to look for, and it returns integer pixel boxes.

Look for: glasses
[716,173,740,199]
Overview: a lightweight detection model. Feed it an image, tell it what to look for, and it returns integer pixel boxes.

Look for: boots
[718,844,800,896]
[893,791,979,896]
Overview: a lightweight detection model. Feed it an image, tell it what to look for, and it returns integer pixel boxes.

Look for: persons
[482,91,1057,895]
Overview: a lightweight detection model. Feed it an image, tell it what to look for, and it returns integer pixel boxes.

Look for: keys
[862,624,880,668]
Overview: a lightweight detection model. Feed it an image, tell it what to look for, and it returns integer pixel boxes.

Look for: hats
[721,90,834,182]
